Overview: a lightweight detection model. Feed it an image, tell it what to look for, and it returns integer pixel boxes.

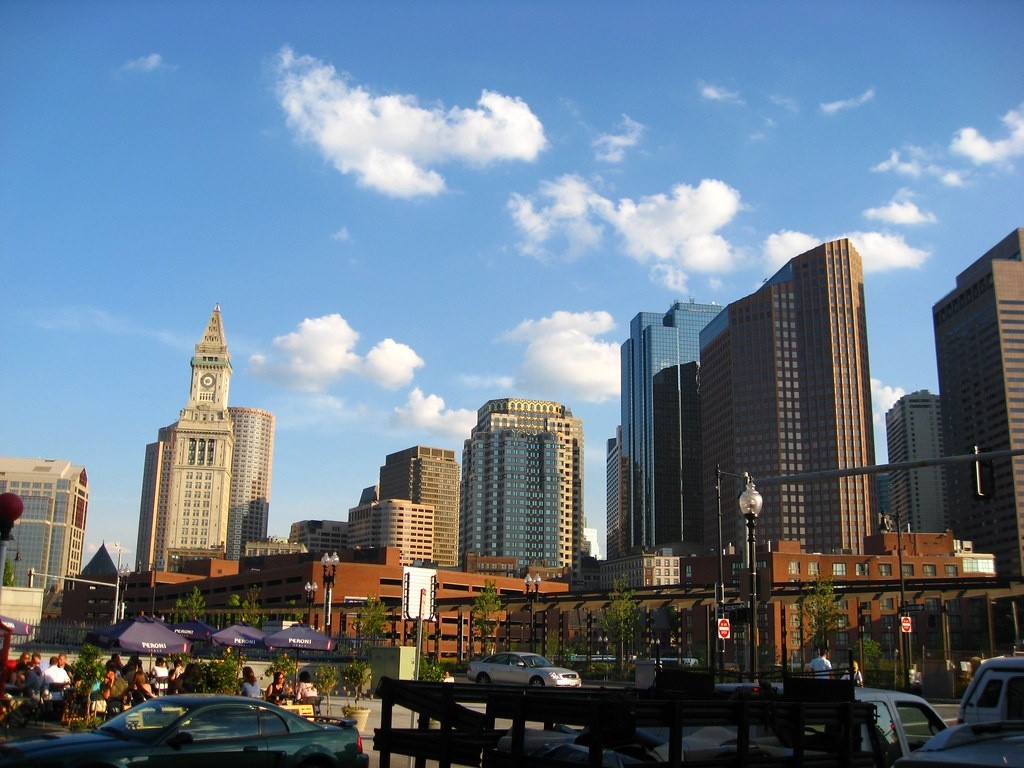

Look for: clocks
[200,373,216,389]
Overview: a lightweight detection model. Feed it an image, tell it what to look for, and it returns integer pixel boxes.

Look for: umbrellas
[264,623,339,671]
[167,617,221,658]
[212,621,270,678]
[0,614,34,636]
[82,612,190,673]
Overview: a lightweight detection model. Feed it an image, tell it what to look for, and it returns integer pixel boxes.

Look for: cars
[372,655,1024,768]
[0,692,370,768]
[467,652,582,688]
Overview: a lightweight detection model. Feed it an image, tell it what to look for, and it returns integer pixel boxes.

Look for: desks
[271,695,296,698]
[5,684,23,698]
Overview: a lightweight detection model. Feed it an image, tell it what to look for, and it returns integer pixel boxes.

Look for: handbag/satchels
[855,671,864,687]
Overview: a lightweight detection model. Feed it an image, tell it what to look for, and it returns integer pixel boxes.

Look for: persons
[265,671,294,705]
[841,660,862,687]
[236,667,260,700]
[971,652,985,678]
[806,649,834,680]
[443,671,454,682]
[0,650,74,738]
[909,664,924,689]
[296,671,320,715]
[75,652,202,721]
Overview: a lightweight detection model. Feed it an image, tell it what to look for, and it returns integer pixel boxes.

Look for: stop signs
[719,619,731,638]
[902,617,912,632]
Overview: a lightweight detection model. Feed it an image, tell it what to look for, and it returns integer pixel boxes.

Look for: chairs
[262,690,324,718]
[146,674,169,696]
[90,690,105,716]
[33,682,66,721]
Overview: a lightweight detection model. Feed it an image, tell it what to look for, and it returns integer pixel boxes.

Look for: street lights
[320,552,340,637]
[138,560,156,618]
[879,508,912,694]
[524,572,541,653]
[112,540,122,626]
[304,581,318,627]
[738,476,763,684]
[597,635,609,661]
[649,639,661,670]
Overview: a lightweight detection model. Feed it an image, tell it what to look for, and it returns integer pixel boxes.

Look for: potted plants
[341,659,376,731]
[70,643,106,733]
[414,654,448,729]
